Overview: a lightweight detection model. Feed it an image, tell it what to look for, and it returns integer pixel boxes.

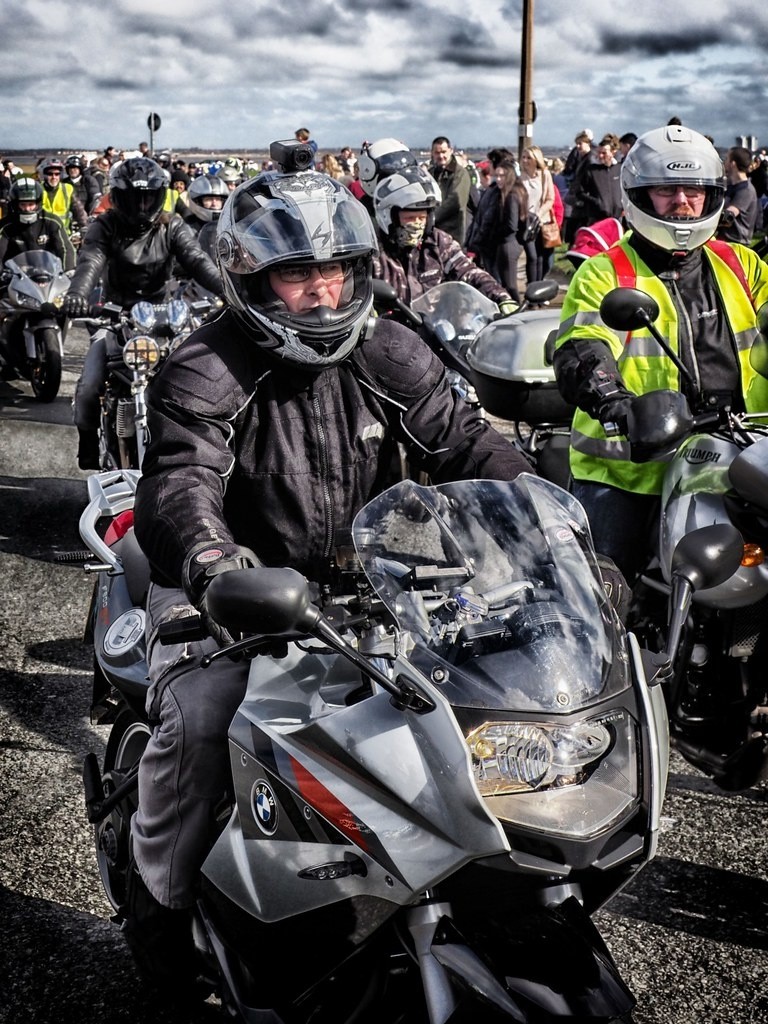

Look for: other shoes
[117,857,197,997]
[78,429,101,471]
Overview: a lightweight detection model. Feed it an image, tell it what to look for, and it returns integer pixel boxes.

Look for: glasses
[45,172,61,176]
[271,257,350,284]
[654,184,706,198]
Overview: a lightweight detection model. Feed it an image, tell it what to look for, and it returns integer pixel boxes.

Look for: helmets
[38,157,64,177]
[9,177,43,224]
[111,157,169,224]
[213,170,379,363]
[357,138,416,199]
[372,164,443,247]
[98,157,109,167]
[186,174,231,223]
[64,156,83,175]
[619,126,727,254]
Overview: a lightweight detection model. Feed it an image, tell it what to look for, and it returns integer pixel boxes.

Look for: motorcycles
[78,467,745,1024]
[0,219,767,472]
[464,286,767,794]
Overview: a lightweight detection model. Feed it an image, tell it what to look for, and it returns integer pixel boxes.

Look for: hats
[169,169,190,192]
[577,127,594,141]
[188,163,196,168]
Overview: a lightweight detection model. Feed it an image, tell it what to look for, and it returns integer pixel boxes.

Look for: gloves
[590,385,642,435]
[61,293,93,319]
[180,540,287,662]
[522,543,632,626]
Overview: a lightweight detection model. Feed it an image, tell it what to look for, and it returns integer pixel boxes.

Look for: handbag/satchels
[539,209,562,248]
[515,207,542,245]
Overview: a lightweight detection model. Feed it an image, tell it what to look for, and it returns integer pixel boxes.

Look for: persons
[67,159,223,470]
[0,118,768,330]
[120,173,560,1002]
[547,123,768,603]
